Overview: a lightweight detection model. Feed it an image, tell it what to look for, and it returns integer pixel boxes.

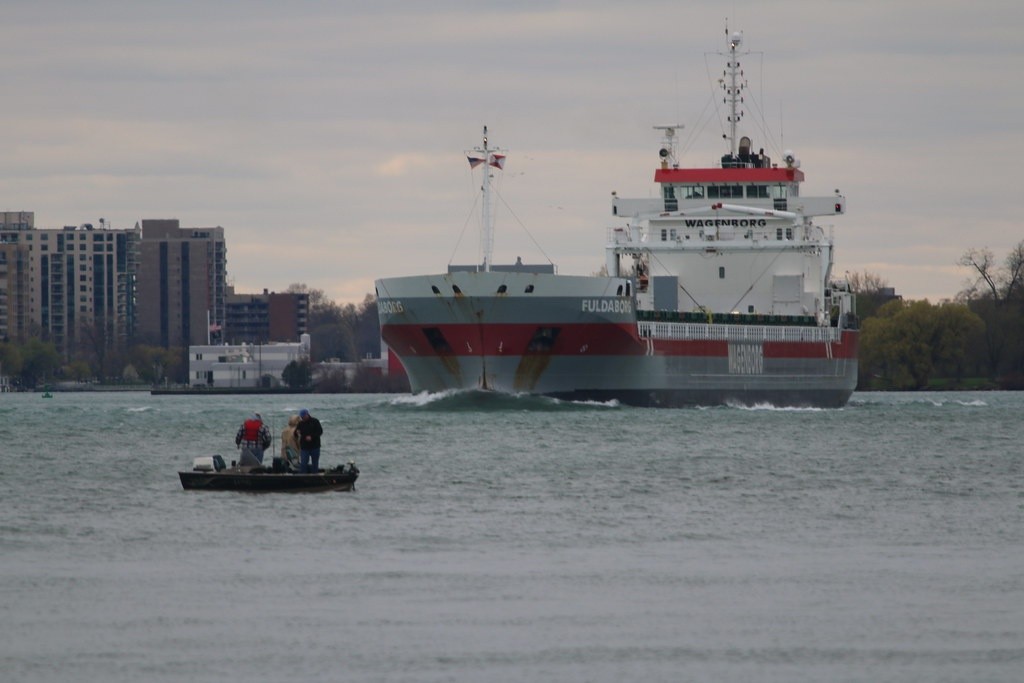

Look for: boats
[176,416,360,493]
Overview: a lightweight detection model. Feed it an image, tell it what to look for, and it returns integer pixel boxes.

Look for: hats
[299,409,309,416]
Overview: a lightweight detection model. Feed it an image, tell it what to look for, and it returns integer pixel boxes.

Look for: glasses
[301,416,304,420]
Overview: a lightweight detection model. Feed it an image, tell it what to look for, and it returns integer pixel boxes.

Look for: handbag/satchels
[285,445,297,460]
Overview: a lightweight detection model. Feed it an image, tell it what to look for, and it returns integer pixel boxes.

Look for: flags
[488,154,506,171]
[467,156,486,170]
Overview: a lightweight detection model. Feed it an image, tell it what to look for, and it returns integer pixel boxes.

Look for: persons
[235,412,272,466]
[281,415,301,470]
[817,309,830,327]
[294,409,323,473]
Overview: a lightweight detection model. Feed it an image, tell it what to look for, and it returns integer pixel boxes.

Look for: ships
[370,17,863,411]
